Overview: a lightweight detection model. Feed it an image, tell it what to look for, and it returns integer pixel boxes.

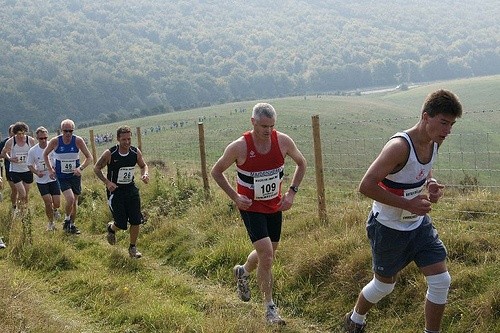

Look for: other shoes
[70,224,81,234]
[0,239,6,248]
[61,219,71,233]
[52,208,61,218]
[47,222,55,232]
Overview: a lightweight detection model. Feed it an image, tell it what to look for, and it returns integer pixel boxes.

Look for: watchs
[79,166,83,170]
[289,186,298,193]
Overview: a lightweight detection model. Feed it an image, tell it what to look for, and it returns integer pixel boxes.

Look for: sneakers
[266,305,285,326]
[128,246,142,258]
[107,224,116,245]
[233,264,252,302]
[344,310,368,333]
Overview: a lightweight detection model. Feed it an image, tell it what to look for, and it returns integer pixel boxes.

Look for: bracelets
[426,177,436,187]
[141,173,149,175]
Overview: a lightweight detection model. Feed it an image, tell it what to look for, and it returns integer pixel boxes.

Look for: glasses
[38,137,47,140]
[62,130,74,133]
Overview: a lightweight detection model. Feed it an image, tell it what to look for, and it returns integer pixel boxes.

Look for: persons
[93,125,150,258]
[211,103,307,325]
[343,90,463,332]
[28,126,61,232]
[1,122,35,213]
[0,124,18,210]
[0,235,7,249]
[44,119,94,234]
